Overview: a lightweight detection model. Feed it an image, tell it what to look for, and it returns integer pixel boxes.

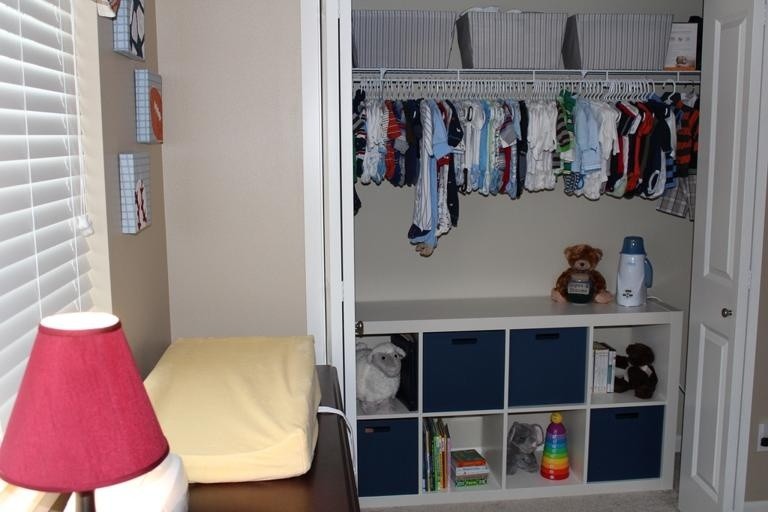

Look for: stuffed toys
[355,340,407,415]
[549,243,614,304]
[615,341,659,400]
[507,421,545,475]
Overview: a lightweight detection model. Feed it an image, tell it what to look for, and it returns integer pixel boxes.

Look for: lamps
[0,313,169,511]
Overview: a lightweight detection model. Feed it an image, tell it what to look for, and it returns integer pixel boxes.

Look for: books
[593,340,617,393]
[423,418,489,492]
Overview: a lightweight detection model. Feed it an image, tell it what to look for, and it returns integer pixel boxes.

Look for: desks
[187,363,363,512]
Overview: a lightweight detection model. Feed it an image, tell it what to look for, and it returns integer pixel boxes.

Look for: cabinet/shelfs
[351,0,705,510]
